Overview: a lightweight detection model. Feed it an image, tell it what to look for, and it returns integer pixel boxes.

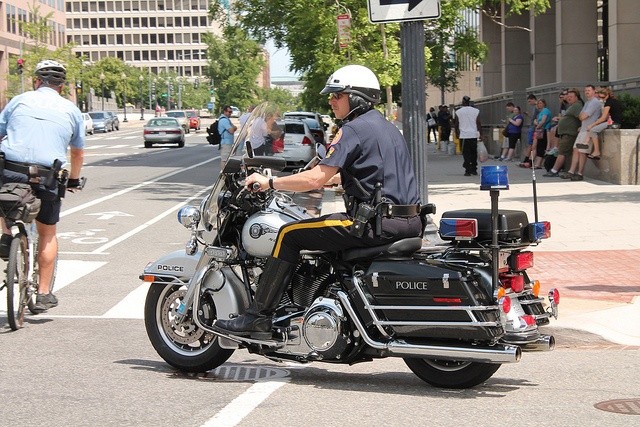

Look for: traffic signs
[367,0,442,24]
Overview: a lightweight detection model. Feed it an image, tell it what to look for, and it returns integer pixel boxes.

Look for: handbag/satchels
[428,112,436,126]
[0,182,41,223]
[273,136,283,153]
[502,123,509,136]
[536,125,544,139]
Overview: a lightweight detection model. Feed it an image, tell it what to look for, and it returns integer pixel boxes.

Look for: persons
[425,105,438,144]
[533,98,551,169]
[216,105,238,171]
[238,104,255,158]
[518,94,539,168]
[548,86,577,155]
[213,64,422,341]
[0,60,87,312]
[543,91,584,179]
[442,105,452,152]
[572,88,584,105]
[587,88,624,160]
[544,93,567,155]
[245,103,273,158]
[503,106,524,162]
[560,84,602,181]
[494,101,516,160]
[454,96,481,176]
[436,104,443,151]
[268,100,283,159]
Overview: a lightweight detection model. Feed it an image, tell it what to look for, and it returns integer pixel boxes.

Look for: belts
[347,204,421,216]
[5,159,51,177]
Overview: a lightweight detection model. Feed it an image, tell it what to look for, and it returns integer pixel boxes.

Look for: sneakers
[543,170,559,176]
[559,171,572,178]
[570,173,583,180]
[0,232,14,260]
[34,291,58,309]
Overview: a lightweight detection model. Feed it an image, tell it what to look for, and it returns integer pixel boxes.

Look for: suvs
[283,112,327,146]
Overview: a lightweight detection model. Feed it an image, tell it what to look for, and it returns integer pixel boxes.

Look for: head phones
[348,93,371,114]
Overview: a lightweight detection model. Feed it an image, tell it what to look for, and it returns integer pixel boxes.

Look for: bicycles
[0,177,87,331]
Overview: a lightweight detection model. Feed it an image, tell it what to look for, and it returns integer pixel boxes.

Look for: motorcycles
[139,102,560,389]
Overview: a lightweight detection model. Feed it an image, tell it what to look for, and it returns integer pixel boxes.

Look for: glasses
[226,110,232,113]
[328,92,343,99]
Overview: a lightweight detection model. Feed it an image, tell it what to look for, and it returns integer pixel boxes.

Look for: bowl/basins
[612,123,621,129]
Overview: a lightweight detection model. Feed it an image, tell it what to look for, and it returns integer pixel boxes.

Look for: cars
[229,107,242,117]
[89,111,119,132]
[263,117,317,163]
[162,110,190,133]
[81,113,94,136]
[185,109,200,130]
[143,117,185,148]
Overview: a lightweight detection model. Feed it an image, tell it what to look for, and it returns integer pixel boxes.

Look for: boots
[213,255,298,340]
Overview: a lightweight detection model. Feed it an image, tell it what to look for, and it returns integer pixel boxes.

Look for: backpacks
[206,116,231,150]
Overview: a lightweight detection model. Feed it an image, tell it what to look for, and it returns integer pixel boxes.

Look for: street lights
[139,74,144,120]
[99,72,106,110]
[121,72,127,121]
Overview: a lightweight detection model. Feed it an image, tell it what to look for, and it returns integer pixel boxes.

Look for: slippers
[576,143,589,149]
[531,165,542,168]
[587,154,600,160]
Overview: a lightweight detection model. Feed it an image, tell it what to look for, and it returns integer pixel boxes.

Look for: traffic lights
[194,77,199,89]
[16,58,25,74]
[16,58,24,73]
[210,85,214,95]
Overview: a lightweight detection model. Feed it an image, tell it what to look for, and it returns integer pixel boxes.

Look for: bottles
[607,115,612,129]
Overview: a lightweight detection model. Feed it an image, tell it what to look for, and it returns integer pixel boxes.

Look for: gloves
[69,179,83,187]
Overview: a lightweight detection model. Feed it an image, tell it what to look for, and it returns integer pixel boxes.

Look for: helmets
[34,59,66,85]
[320,64,380,121]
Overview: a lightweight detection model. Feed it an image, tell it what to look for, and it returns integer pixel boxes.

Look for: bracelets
[510,119,513,123]
[268,175,275,191]
